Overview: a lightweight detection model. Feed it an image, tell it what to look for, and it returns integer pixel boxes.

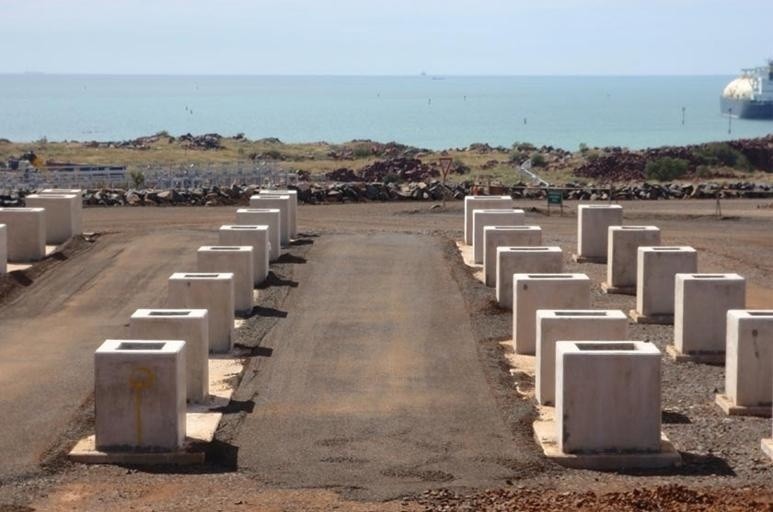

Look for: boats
[719,60,773,120]
